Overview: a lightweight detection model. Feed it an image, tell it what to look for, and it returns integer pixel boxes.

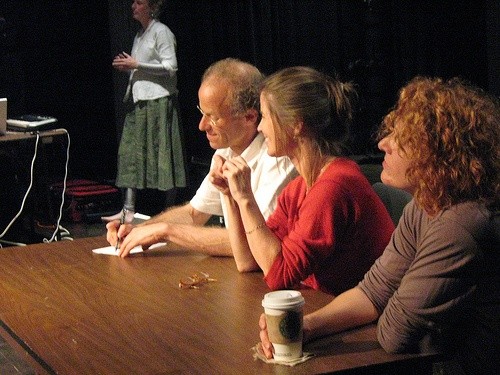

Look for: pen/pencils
[115,208,125,252]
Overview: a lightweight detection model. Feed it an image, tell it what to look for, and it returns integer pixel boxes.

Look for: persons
[259,78,500,375]
[209,67,395,295]
[101,0,186,222]
[105,60,300,258]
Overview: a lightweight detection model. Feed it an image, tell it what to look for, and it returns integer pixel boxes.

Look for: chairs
[372,182,413,228]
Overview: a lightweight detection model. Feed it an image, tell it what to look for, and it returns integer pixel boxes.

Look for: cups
[262,290,306,363]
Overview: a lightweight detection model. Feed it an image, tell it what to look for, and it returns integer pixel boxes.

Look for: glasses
[197,103,250,127]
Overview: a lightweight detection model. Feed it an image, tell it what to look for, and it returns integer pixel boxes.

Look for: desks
[0,236,444,375]
[0,129,67,143]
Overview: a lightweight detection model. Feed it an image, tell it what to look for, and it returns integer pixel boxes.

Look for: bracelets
[247,224,265,234]
